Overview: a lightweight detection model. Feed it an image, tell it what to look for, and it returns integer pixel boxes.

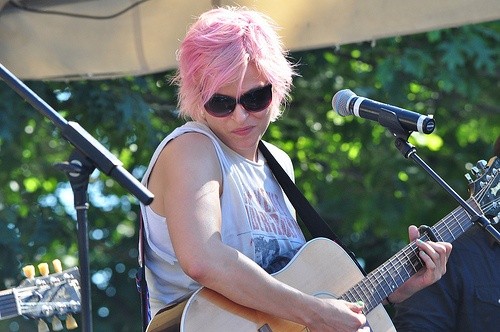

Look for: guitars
[144,151,499,332]
[1,258,81,332]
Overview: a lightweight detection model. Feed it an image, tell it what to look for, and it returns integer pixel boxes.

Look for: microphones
[331,88,436,134]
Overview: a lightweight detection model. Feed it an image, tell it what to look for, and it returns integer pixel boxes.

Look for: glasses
[199,84,272,118]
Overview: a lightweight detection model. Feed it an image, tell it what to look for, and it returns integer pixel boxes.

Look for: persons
[141,8,454,332]
